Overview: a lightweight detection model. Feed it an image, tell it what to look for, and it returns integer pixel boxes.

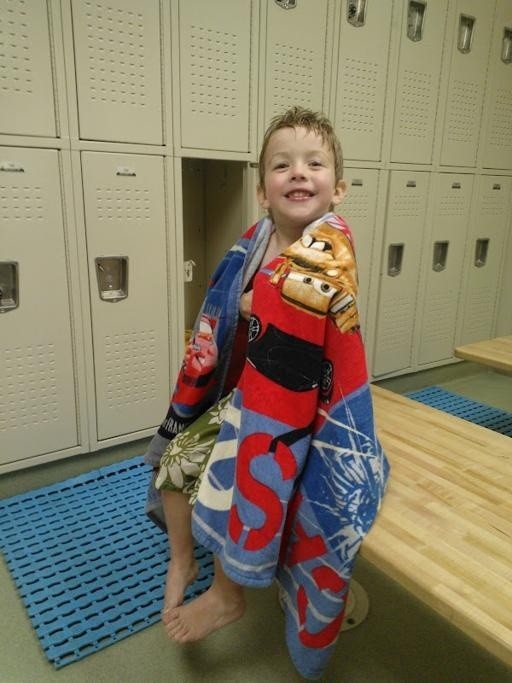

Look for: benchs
[448,330,511,371]
[262,384,511,666]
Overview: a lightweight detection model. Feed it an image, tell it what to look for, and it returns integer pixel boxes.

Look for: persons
[138,105,393,683]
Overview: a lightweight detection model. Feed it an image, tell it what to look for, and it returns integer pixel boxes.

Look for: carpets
[0,383,512,668]
